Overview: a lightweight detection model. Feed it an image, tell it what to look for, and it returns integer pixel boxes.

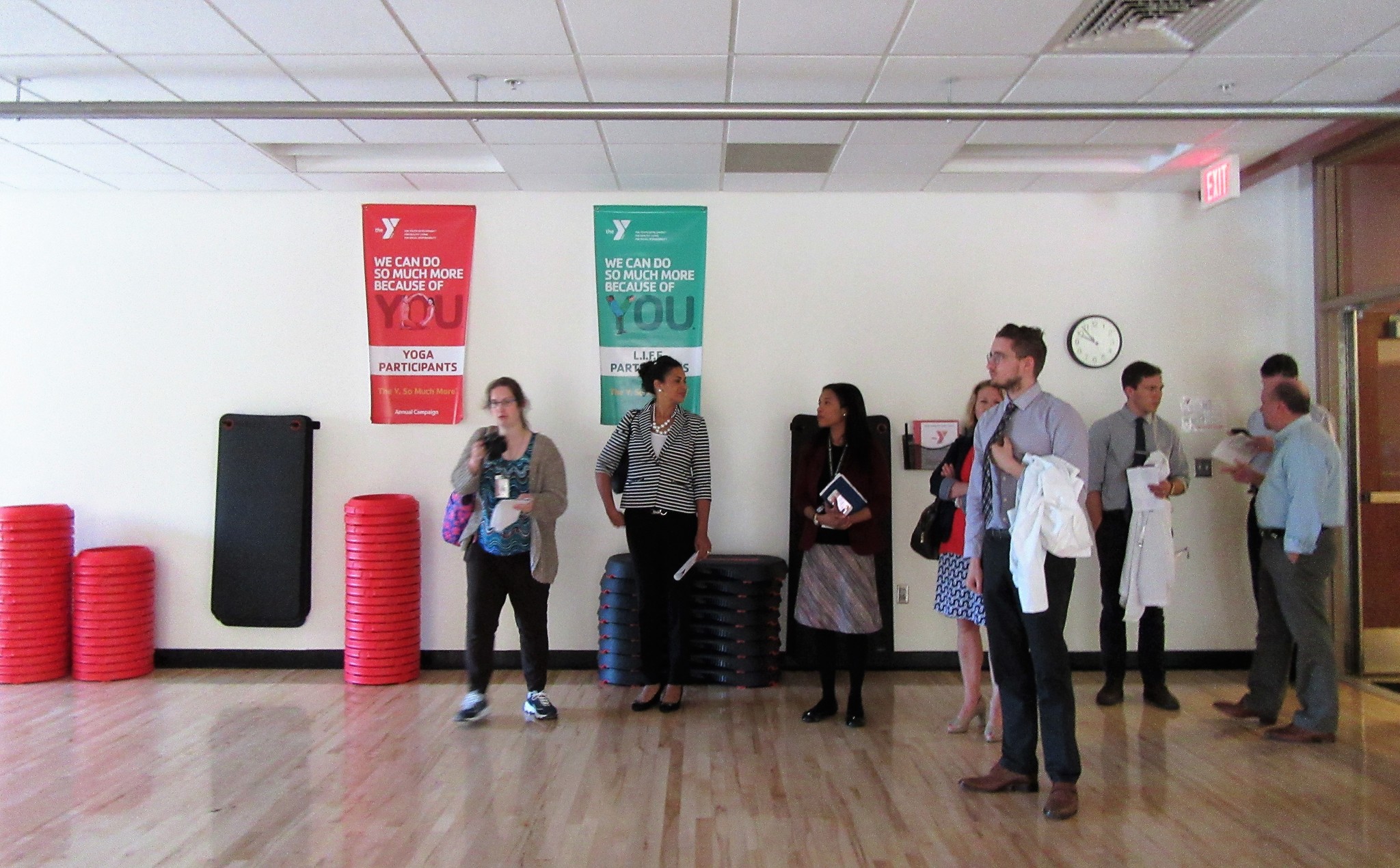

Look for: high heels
[947,695,986,732]
[659,684,683,712]
[983,706,1003,742]
[631,682,665,711]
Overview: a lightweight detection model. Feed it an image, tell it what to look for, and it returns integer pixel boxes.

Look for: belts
[986,527,1011,539]
[1258,528,1286,539]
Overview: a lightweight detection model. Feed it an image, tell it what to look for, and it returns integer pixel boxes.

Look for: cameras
[481,432,508,459]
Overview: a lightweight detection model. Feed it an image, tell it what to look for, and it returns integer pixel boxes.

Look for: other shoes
[1096,681,1124,705]
[1143,684,1180,711]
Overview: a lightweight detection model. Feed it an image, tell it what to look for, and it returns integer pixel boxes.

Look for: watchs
[1169,481,1175,497]
[813,513,819,526]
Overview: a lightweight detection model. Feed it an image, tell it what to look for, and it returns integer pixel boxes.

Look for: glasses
[986,353,1026,363]
[490,398,519,408]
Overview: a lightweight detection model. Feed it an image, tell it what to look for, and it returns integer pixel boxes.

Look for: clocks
[1066,314,1123,369]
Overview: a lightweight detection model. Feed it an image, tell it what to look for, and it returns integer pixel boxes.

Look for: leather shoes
[845,704,866,726]
[1042,782,1079,820]
[958,762,1038,794]
[1263,724,1337,744]
[1212,701,1277,725]
[802,701,838,722]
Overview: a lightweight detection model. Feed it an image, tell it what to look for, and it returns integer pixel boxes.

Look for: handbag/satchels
[611,409,641,495]
[442,427,487,547]
[909,497,956,560]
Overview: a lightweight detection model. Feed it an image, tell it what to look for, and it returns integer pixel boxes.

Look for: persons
[1213,378,1347,745]
[1228,353,1339,689]
[791,383,883,727]
[1092,361,1188,711]
[958,324,1090,819]
[595,356,712,714]
[450,378,567,724]
[929,380,1007,743]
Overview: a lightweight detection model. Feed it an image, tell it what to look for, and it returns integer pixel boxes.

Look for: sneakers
[452,689,489,722]
[523,689,558,720]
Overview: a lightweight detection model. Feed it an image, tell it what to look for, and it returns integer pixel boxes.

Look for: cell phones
[826,488,854,517]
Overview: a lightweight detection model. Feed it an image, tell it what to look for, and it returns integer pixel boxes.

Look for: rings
[706,550,711,555]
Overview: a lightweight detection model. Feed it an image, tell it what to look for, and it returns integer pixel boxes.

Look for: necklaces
[652,401,679,435]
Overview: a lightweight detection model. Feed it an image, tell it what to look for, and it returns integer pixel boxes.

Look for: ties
[1123,417,1147,526]
[981,402,1017,526]
